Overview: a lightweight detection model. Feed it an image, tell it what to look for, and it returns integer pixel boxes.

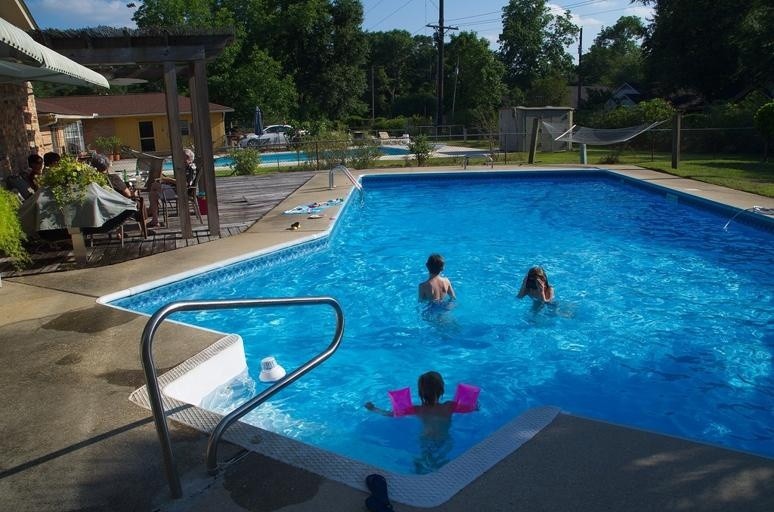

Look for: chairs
[7,149,205,247]
[379,132,392,145]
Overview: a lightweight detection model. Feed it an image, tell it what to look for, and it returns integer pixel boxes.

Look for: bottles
[257,356,286,382]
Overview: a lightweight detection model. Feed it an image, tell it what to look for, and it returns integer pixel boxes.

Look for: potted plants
[95,135,121,162]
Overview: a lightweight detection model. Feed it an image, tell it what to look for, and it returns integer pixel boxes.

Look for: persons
[42,151,61,175]
[25,153,44,191]
[144,147,197,229]
[360,369,481,475]
[414,253,458,328]
[90,153,159,240]
[515,264,575,328]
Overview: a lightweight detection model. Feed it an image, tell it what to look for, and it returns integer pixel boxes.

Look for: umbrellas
[254,105,265,146]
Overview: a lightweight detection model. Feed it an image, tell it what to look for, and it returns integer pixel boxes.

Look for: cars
[239,125,311,148]
